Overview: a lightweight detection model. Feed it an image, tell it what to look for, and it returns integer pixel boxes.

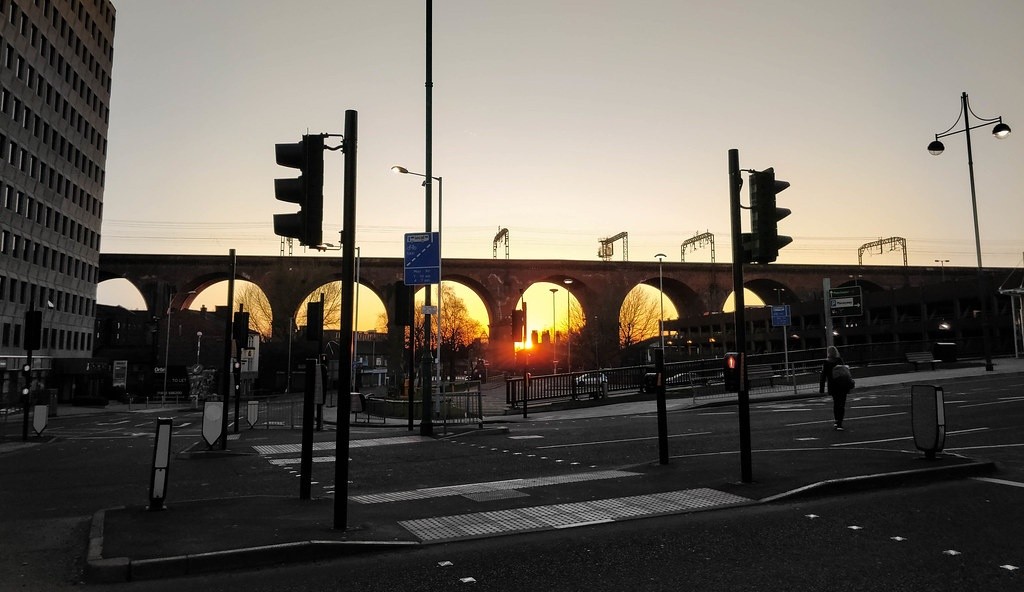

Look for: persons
[819,346,855,431]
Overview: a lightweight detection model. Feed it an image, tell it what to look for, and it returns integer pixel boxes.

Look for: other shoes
[834,423,838,426]
[837,427,844,430]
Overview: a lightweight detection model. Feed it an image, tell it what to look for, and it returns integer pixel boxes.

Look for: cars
[464,376,472,381]
[665,373,698,384]
[574,372,608,387]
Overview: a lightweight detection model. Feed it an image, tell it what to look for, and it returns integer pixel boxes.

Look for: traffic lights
[21,364,32,376]
[233,361,241,370]
[21,387,30,397]
[749,168,793,262]
[510,309,523,342]
[274,134,324,246]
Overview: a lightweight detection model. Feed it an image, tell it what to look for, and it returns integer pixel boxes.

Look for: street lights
[850,275,863,344]
[773,288,785,307]
[196,332,203,364]
[550,289,558,374]
[935,259,950,267]
[164,291,197,394]
[564,279,573,373]
[392,166,441,417]
[287,316,308,394]
[654,253,667,364]
[928,92,1011,267]
[324,243,355,393]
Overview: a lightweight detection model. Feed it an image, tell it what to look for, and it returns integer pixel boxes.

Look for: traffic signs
[771,305,792,327]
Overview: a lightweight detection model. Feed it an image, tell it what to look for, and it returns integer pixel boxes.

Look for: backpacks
[832,364,855,394]
